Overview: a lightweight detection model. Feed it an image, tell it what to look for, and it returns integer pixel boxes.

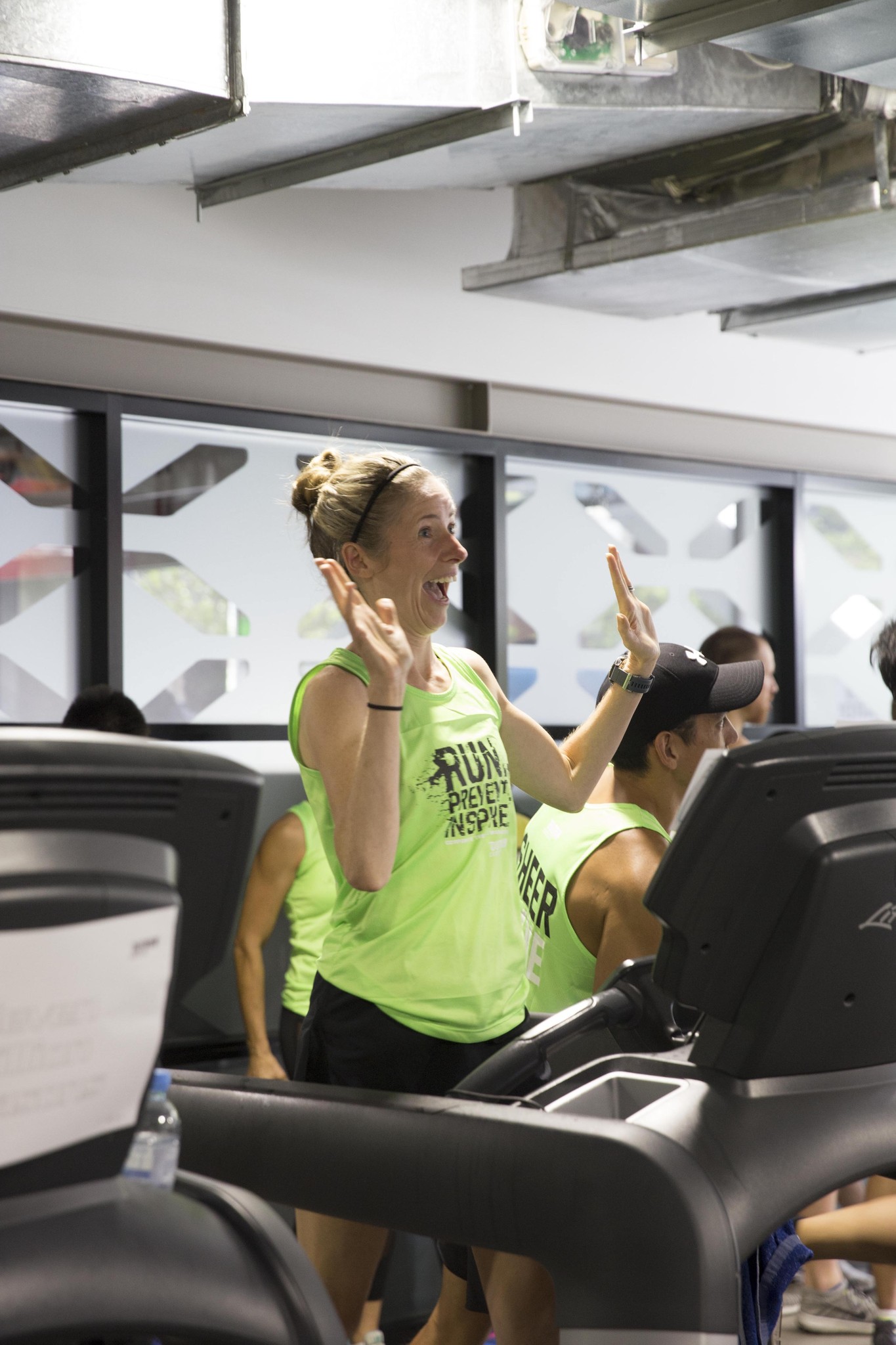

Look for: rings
[628,586,634,591]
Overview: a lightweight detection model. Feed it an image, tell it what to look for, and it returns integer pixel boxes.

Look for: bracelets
[367,702,403,711]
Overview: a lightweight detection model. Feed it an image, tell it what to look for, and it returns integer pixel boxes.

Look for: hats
[595,643,764,751]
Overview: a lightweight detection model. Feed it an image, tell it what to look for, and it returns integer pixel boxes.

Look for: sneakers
[798,1276,881,1334]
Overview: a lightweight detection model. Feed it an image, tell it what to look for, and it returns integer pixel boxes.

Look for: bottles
[118,1068,180,1191]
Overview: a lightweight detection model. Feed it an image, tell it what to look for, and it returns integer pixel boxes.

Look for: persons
[473,643,764,1345]
[799,617,896,1345]
[699,625,778,747]
[233,801,466,1345]
[288,448,659,1345]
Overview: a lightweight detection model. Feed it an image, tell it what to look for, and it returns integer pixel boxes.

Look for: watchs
[608,654,655,693]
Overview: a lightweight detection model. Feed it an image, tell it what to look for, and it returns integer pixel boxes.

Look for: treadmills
[2,725,353,1345]
[151,723,895,1344]
